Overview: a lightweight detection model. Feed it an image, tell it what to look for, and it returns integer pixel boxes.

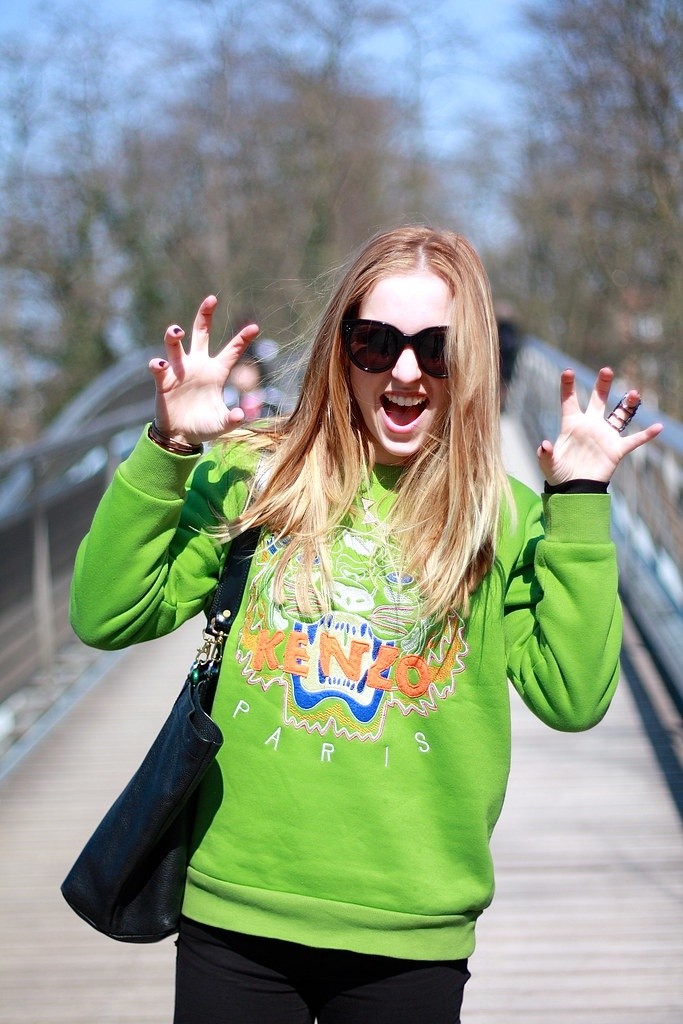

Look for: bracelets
[147,421,205,456]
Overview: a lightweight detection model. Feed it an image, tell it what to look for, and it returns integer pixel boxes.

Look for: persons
[499,300,521,413]
[68,225,664,1020]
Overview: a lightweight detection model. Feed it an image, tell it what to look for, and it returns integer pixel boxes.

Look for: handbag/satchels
[62,498,264,944]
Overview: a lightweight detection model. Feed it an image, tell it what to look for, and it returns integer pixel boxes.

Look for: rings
[608,390,640,432]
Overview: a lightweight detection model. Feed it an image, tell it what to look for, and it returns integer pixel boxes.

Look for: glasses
[343,318,455,382]
